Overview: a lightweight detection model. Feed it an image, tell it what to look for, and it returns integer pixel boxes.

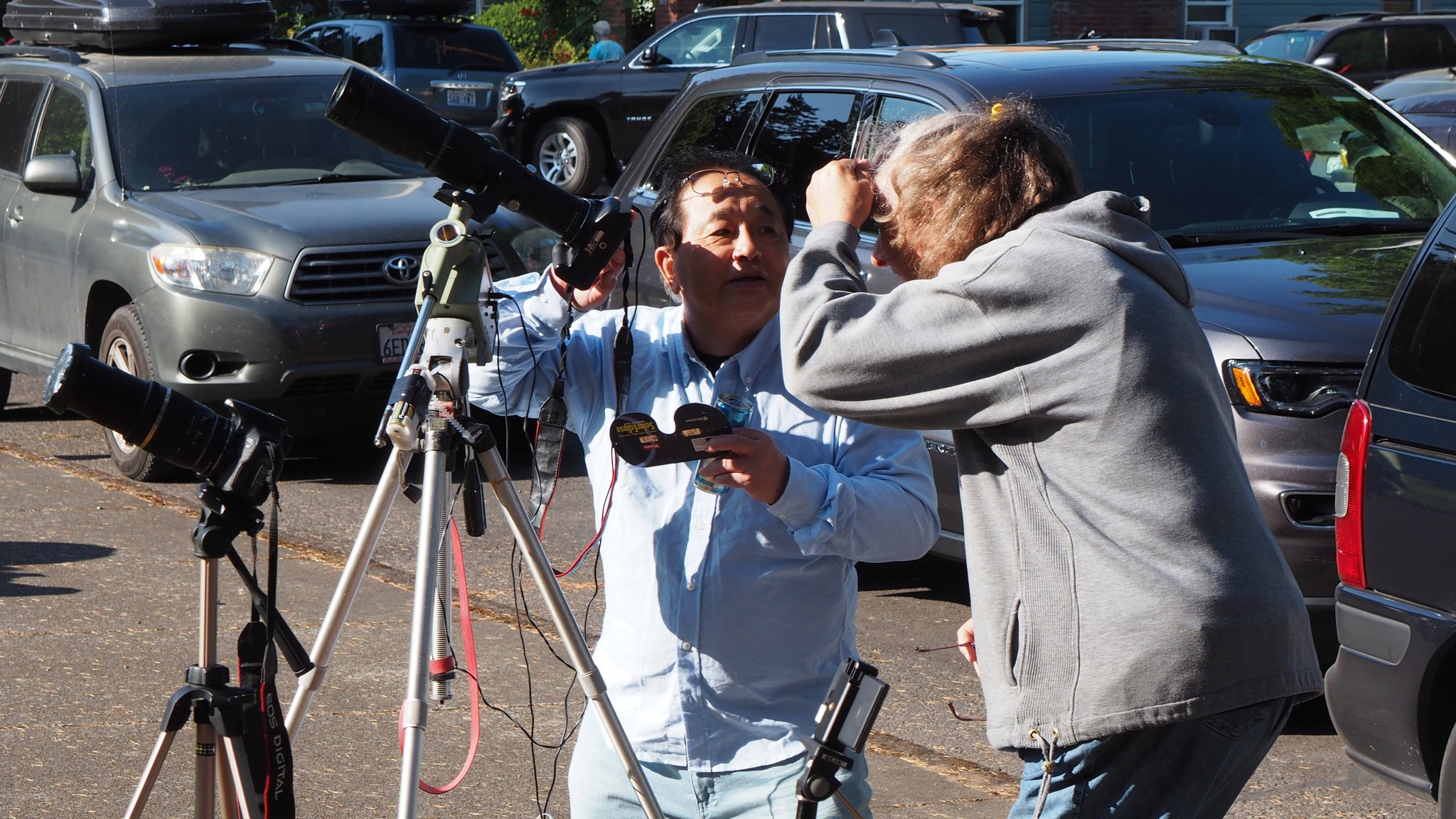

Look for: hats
[594,20,611,35]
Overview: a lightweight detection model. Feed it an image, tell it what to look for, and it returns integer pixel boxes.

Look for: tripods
[278,196,669,819]
[115,506,267,819]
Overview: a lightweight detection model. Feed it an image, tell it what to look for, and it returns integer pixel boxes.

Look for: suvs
[0,0,560,485]
[490,0,1006,198]
[602,35,1456,653]
[286,0,525,131]
[1323,188,1456,819]
[1241,12,1456,191]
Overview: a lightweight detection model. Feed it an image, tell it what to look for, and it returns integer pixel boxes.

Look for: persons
[781,106,1323,819]
[588,20,625,62]
[467,152,941,819]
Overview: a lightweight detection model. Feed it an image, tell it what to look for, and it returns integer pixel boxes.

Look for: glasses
[675,162,782,195]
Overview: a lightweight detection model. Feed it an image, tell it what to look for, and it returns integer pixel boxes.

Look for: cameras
[811,654,891,753]
[39,343,293,509]
[323,66,632,290]
